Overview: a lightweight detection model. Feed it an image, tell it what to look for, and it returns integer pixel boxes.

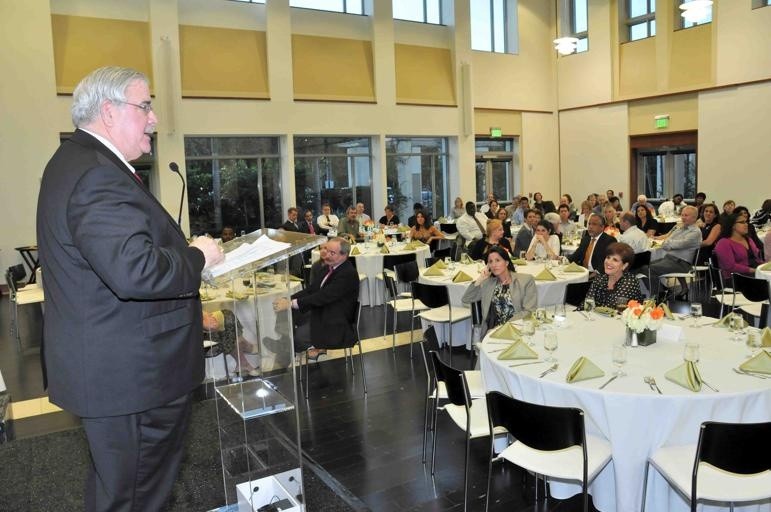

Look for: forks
[642,374,664,394]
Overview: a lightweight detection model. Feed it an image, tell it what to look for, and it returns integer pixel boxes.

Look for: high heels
[233,369,261,382]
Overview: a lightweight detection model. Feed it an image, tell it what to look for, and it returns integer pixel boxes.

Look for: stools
[203,341,232,397]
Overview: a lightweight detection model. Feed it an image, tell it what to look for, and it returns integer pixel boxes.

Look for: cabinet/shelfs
[201,229,327,512]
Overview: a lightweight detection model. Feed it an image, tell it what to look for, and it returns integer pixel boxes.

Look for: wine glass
[443,250,569,283]
[333,218,413,252]
[519,303,763,376]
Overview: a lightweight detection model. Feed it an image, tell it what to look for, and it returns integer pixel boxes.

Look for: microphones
[168,161,186,229]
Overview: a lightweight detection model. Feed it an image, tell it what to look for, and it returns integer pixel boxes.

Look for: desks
[202,271,303,379]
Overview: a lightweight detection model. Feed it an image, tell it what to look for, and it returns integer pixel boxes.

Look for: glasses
[110,99,153,113]
[735,220,750,224]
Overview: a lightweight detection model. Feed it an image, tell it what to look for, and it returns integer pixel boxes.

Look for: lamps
[553,0,580,56]
[679,1,715,25]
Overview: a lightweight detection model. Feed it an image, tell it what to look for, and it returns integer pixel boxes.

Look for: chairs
[298,221,447,398]
[420,200,771,512]
[6,244,44,339]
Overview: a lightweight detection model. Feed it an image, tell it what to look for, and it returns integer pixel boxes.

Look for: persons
[36,65,225,512]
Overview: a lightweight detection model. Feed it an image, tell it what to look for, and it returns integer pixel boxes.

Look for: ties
[472,214,485,234]
[326,216,332,227]
[309,223,315,234]
[582,238,596,268]
